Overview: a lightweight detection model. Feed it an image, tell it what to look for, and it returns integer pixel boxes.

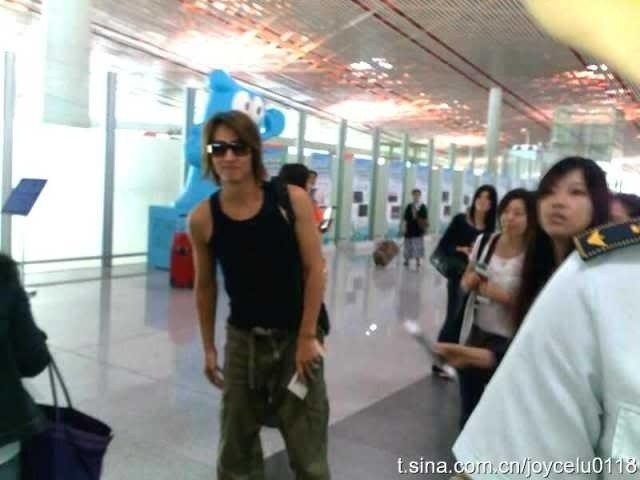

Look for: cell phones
[286,371,309,401]
[474,260,489,284]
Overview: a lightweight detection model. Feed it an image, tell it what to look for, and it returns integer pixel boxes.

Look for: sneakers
[436,363,456,381]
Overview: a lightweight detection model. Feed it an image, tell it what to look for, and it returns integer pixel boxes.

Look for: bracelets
[298,332,318,341]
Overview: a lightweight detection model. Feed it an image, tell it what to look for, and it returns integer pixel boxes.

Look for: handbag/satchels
[21,332,116,479]
[430,246,469,279]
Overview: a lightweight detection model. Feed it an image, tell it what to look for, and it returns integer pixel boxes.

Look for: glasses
[202,138,253,160]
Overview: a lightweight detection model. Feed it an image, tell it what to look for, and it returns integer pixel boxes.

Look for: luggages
[369,240,408,268]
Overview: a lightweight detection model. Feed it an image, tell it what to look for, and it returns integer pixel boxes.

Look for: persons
[274,162,330,348]
[185,112,334,479]
[428,153,639,437]
[395,188,430,270]
[449,217,640,480]
[308,168,325,227]
[0,254,54,480]
